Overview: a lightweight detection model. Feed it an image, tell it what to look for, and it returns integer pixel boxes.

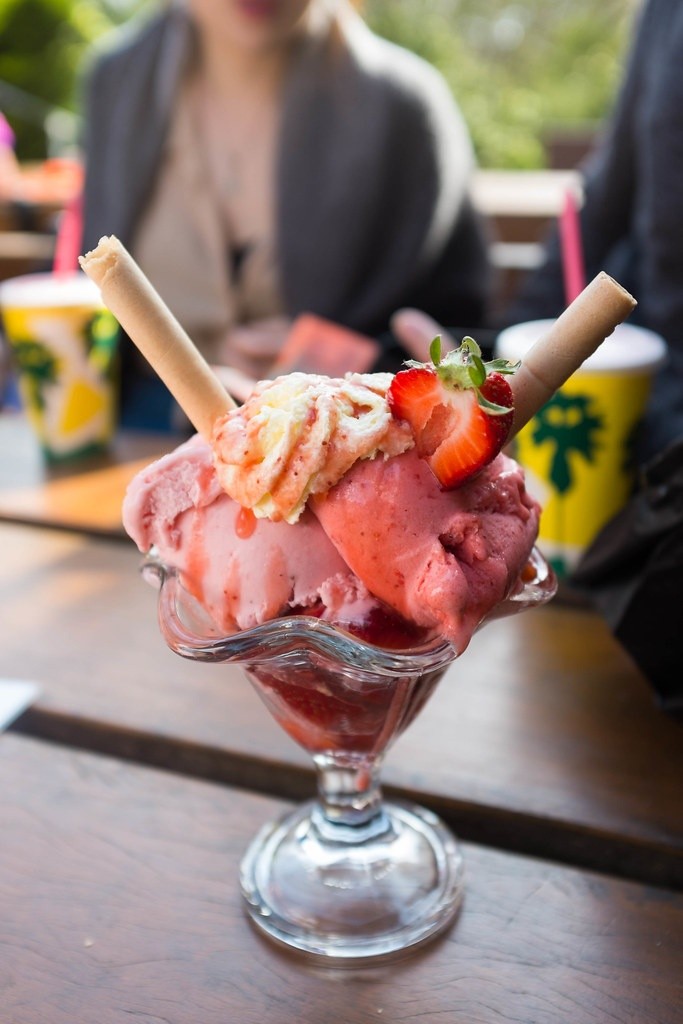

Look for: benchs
[1,162,579,302]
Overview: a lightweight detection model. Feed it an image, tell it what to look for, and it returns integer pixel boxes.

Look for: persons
[390,1,683,460]
[75,1,489,433]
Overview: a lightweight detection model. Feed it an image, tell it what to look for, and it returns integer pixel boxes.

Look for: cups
[498,317,664,580]
[1,272,122,469]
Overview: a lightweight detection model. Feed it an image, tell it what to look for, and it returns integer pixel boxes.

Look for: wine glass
[137,543,556,958]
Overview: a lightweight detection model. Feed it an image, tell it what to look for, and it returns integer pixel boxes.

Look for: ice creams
[78,232,638,762]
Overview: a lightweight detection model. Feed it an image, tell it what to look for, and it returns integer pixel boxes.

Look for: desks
[0,413,683,1024]
